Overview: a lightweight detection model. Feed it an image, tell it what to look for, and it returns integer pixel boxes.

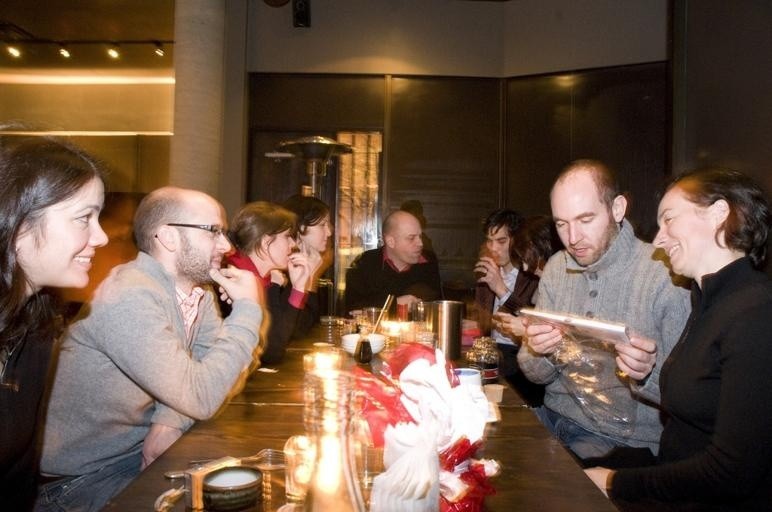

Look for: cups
[362,307,389,338]
[400,321,434,344]
[474,246,497,284]
[320,316,341,344]
[361,448,384,490]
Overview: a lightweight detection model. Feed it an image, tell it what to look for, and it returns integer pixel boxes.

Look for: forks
[188,448,285,466]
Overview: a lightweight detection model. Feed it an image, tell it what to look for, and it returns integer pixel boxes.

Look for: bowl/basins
[342,335,386,357]
[201,466,264,511]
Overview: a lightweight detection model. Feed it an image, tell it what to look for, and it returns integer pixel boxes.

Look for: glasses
[155,222,227,234]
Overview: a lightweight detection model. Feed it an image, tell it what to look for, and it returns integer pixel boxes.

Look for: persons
[346,211,443,313]
[0,133,108,512]
[517,159,691,468]
[285,196,332,333]
[581,165,772,512]
[40,185,264,512]
[213,199,311,371]
[492,214,566,343]
[474,209,539,379]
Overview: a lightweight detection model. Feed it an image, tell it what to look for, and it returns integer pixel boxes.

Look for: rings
[482,269,488,275]
